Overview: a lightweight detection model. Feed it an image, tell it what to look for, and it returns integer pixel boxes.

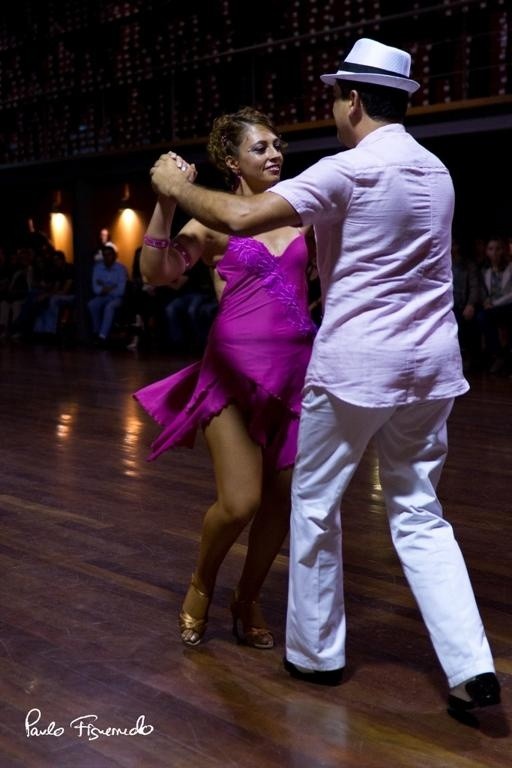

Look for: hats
[320,35,421,95]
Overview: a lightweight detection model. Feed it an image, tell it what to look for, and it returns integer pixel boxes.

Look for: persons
[139,105,318,650]
[0,230,512,375]
[147,37,502,718]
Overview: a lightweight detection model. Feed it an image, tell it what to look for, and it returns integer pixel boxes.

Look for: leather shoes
[448,671,502,716]
[280,654,347,685]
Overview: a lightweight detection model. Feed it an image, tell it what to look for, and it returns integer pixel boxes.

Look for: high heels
[229,591,275,648]
[179,574,213,646]
